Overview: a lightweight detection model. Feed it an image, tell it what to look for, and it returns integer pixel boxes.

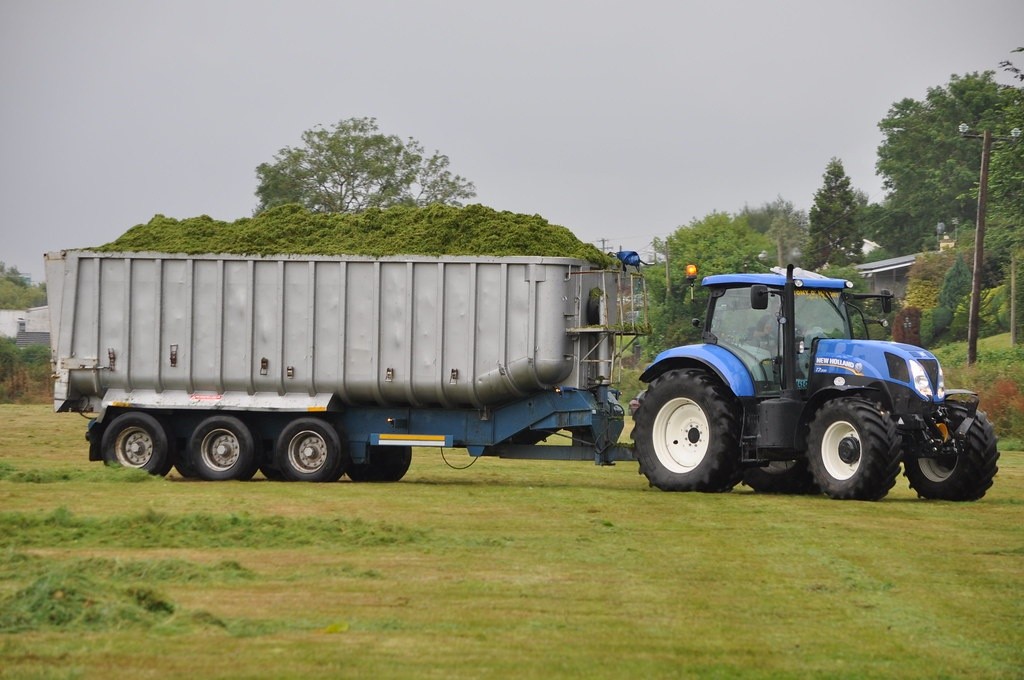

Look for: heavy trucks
[42,250,1000,502]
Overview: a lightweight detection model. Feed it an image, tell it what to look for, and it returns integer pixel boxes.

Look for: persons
[753,299,780,337]
